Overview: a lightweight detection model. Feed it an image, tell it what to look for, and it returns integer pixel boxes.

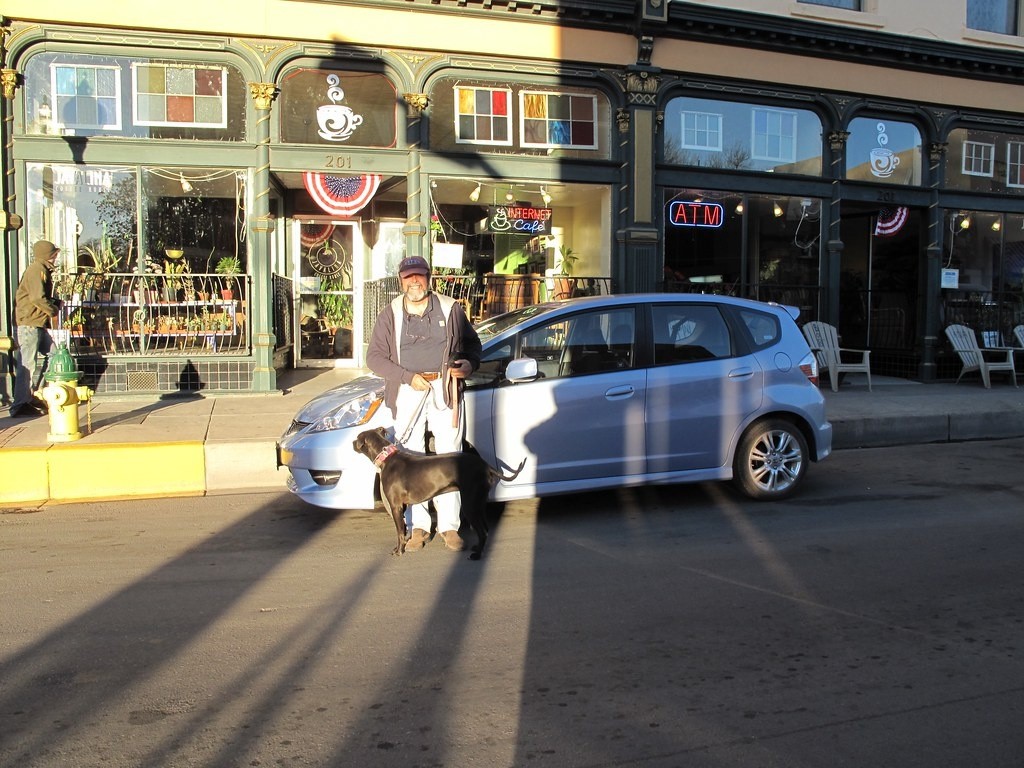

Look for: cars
[272,290,834,526]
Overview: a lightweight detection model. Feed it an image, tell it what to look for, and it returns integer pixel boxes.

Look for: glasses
[406,315,429,340]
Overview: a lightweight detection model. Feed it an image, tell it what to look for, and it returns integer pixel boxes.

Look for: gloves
[50,306,58,317]
[54,299,63,308]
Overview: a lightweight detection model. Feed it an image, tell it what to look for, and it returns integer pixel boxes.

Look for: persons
[366,254,483,551]
[8,241,66,419]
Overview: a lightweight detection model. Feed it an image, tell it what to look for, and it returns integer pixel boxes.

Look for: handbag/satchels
[448,351,466,393]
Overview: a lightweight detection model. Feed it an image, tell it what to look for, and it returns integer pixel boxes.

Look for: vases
[430,230,438,242]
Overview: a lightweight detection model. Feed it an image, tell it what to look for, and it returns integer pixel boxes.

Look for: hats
[34,241,60,260]
[399,256,430,278]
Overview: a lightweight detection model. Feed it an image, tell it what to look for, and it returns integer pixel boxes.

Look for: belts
[423,374,442,381]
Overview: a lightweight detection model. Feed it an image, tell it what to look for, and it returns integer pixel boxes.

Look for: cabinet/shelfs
[58,300,237,349]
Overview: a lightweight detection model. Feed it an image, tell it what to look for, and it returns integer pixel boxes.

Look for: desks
[986,347,1024,386]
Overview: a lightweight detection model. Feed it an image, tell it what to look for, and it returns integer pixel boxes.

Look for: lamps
[539,186,553,204]
[960,215,972,228]
[506,184,514,200]
[773,199,783,217]
[470,183,481,202]
[735,199,744,215]
[695,196,706,202]
[164,245,184,267]
[992,217,1001,231]
[179,172,193,194]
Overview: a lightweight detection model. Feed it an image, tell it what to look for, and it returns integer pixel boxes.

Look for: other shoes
[11,405,41,418]
[30,398,42,408]
[440,530,464,551]
[405,529,431,552]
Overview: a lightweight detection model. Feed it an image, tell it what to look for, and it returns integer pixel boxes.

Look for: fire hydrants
[35,342,95,443]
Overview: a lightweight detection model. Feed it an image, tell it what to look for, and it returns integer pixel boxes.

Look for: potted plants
[317,240,354,335]
[53,221,241,303]
[553,244,580,283]
[63,307,235,333]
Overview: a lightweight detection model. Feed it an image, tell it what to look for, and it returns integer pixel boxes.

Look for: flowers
[430,215,445,234]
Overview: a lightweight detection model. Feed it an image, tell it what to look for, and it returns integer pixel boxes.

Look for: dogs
[352,426,525,562]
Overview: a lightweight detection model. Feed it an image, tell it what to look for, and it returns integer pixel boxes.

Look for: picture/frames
[518,261,546,276]
[531,237,539,254]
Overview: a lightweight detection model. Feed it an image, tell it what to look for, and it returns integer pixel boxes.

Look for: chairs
[1013,325,1024,348]
[575,324,633,374]
[803,321,872,393]
[945,324,1018,388]
